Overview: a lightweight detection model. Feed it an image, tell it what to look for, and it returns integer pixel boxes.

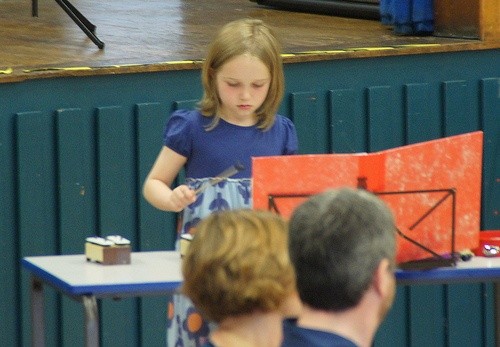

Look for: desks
[22,249,500,346]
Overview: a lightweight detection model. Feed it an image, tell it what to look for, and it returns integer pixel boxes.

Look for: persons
[181,207,302,347]
[287,184,396,347]
[143,17,299,347]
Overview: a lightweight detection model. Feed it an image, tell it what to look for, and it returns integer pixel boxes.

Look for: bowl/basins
[471,230,500,257]
